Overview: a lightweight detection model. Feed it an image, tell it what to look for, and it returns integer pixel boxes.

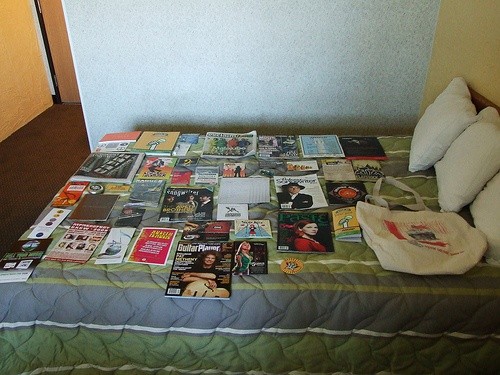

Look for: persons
[216,138,227,155]
[181,250,219,296]
[276,182,313,209]
[151,159,165,168]
[226,138,238,156]
[249,224,256,236]
[207,138,218,155]
[237,138,251,156]
[195,190,214,220]
[158,195,177,221]
[290,220,326,252]
[172,193,198,220]
[232,241,253,275]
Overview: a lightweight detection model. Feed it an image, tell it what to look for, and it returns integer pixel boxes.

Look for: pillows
[469,172,500,266]
[408,76,480,173]
[435,106,499,213]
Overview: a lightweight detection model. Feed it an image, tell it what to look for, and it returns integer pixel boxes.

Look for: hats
[282,183,305,190]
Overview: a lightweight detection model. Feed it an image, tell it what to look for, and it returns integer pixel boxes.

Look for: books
[0,130,388,300]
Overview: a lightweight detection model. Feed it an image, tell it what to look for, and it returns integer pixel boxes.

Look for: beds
[0,87,500,375]
